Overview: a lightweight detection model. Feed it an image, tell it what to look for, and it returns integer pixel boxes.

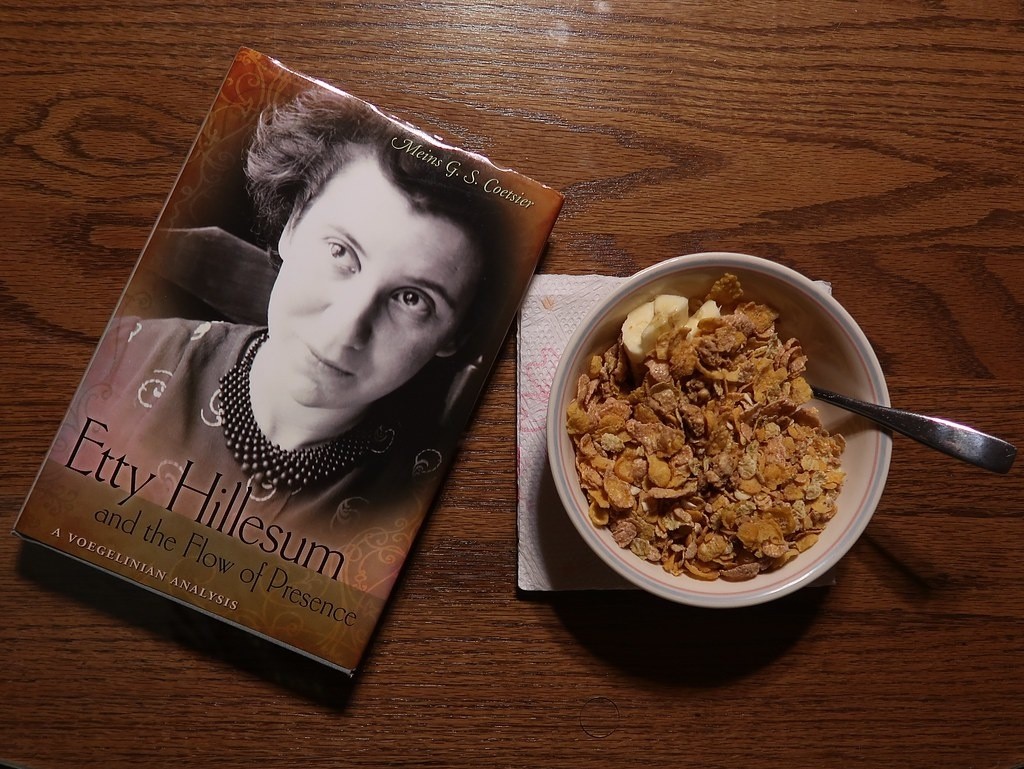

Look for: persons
[53,97,508,593]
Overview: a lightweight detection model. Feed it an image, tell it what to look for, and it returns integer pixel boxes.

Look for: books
[12,45,568,688]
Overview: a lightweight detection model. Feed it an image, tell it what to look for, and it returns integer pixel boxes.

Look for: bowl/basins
[547,252,892,608]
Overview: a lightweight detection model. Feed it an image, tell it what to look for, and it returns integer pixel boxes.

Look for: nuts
[566,274,846,581]
